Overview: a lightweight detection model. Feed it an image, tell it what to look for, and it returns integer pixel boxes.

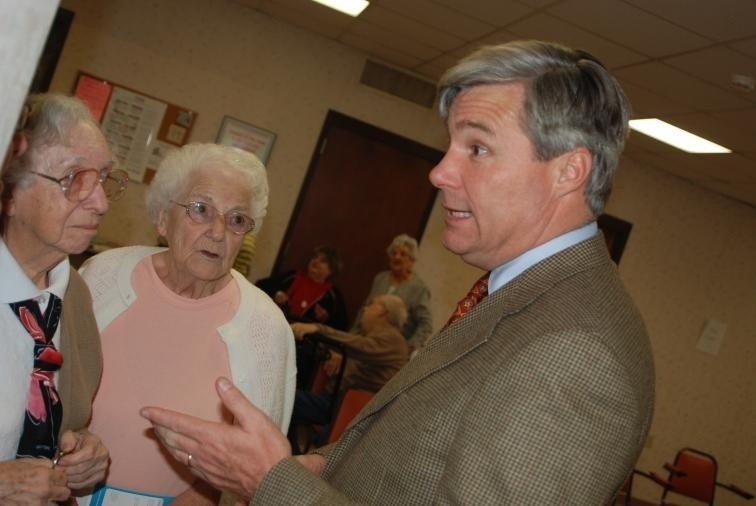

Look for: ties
[438,269,489,334]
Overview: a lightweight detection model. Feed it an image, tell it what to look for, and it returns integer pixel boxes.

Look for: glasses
[21,166,130,203]
[168,197,255,236]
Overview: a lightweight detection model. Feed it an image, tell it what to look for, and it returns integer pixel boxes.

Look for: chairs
[661,447,756,506]
[610,468,670,505]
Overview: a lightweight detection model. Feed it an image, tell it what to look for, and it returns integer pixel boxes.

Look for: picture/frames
[213,111,277,168]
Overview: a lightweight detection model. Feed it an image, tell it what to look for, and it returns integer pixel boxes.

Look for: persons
[0,92,130,505]
[140,38,657,506]
[76,141,299,505]
[287,294,409,454]
[254,243,347,384]
[352,232,433,360]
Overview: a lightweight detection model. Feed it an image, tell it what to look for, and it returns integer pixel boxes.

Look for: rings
[188,454,192,467]
